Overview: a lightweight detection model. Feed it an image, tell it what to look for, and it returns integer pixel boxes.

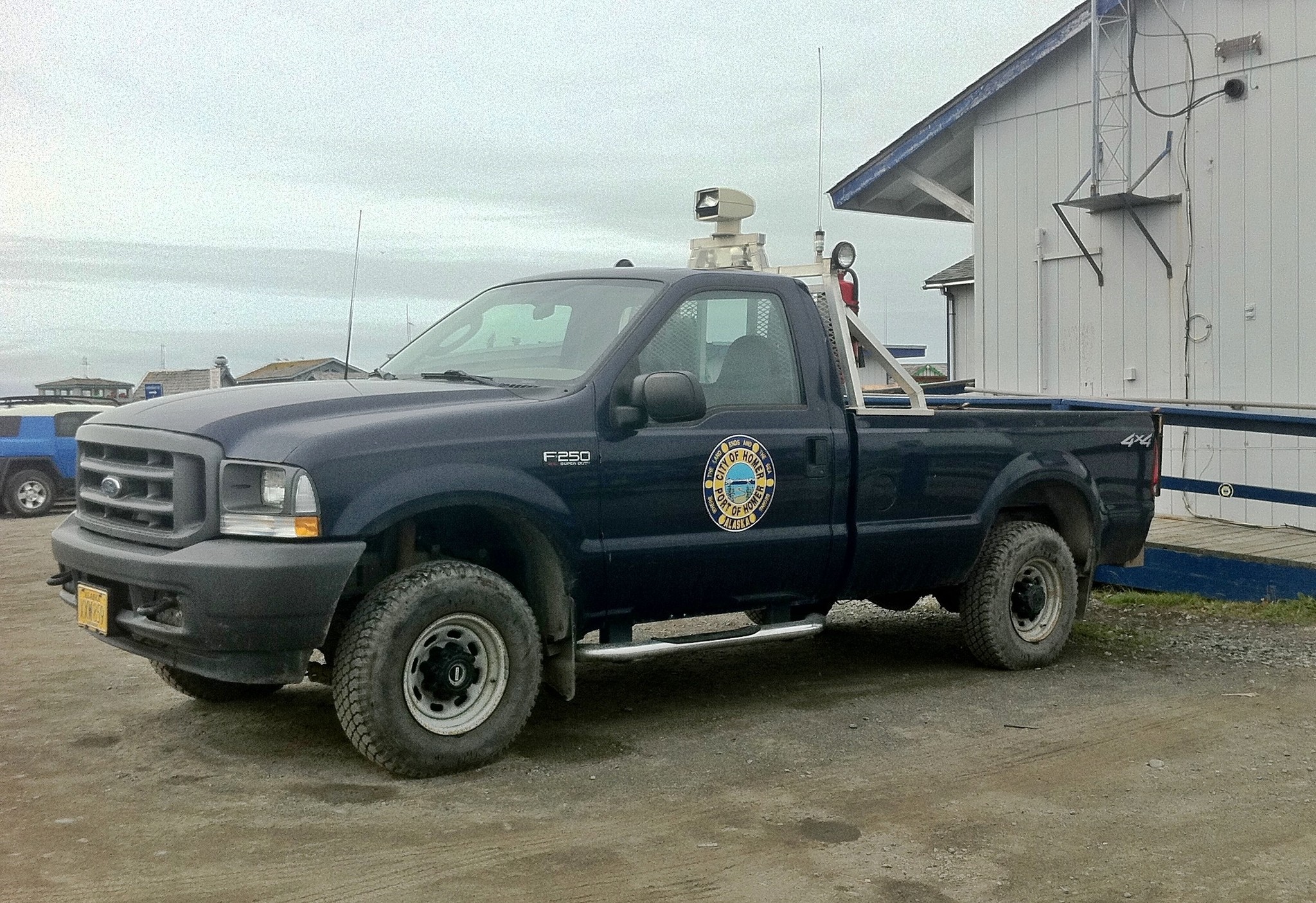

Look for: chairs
[699,335,783,407]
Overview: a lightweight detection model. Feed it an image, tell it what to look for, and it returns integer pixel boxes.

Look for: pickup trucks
[49,253,1172,776]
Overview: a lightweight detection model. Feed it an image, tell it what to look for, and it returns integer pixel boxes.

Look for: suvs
[0,396,129,515]
